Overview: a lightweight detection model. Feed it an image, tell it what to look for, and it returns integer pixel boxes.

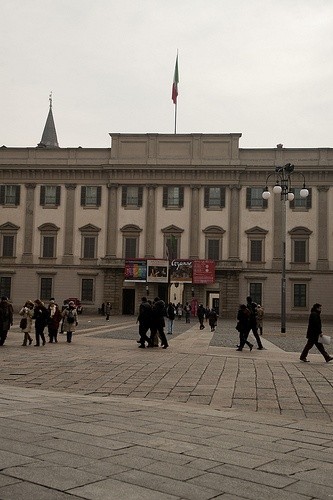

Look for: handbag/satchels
[20,318,27,329]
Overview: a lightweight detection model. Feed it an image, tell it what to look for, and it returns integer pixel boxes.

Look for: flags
[171,53,179,104]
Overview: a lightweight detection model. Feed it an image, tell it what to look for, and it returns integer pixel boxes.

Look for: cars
[62,298,82,314]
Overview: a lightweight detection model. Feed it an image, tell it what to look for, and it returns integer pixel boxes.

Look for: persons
[300,303,333,363]
[101,301,113,320]
[235,296,264,351]
[19,298,80,346]
[135,296,192,349]
[0,295,14,346]
[197,304,217,332]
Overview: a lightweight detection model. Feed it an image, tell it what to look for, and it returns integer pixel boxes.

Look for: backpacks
[67,312,74,323]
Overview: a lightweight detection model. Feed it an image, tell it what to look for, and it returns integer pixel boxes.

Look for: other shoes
[138,346,145,348]
[28,339,33,345]
[147,345,158,347]
[250,344,253,351]
[300,358,310,362]
[325,357,333,362]
[256,347,264,350]
[34,344,40,346]
[161,342,168,349]
[137,340,140,343]
[42,341,46,346]
[235,348,243,351]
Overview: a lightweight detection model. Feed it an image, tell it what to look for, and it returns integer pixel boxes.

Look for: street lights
[261,162,310,332]
